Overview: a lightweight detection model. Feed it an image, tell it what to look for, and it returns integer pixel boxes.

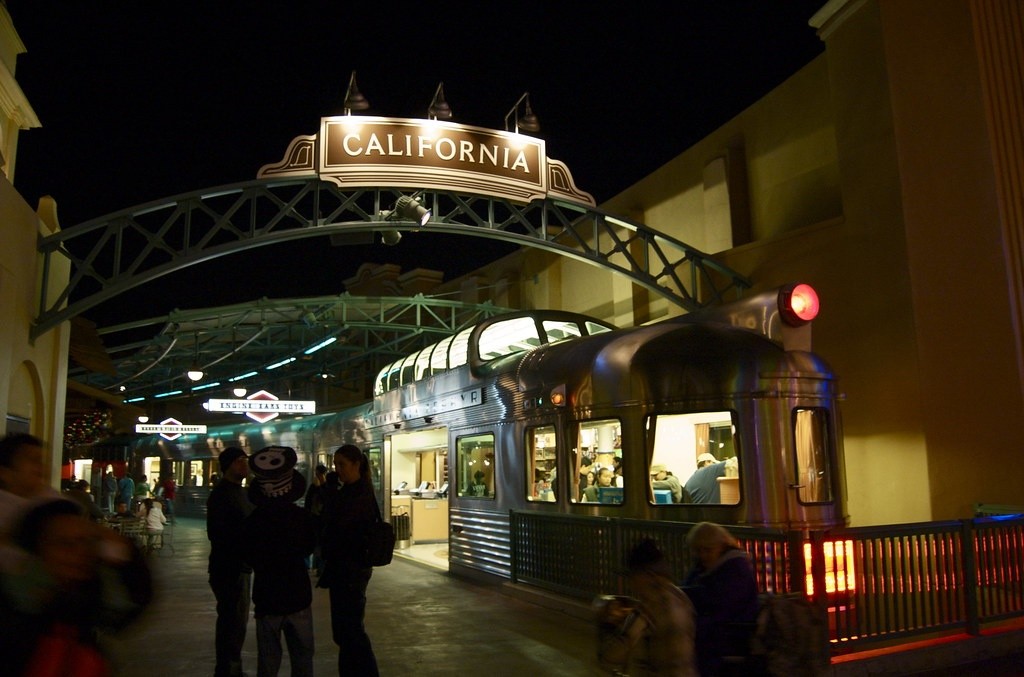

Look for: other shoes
[171,519,177,526]
[161,522,168,525]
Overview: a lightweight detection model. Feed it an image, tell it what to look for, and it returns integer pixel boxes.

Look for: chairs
[116,516,178,557]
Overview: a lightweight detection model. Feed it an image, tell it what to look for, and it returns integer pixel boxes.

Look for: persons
[599,541,697,677]
[152,472,179,524]
[60,478,104,522]
[207,443,253,677]
[678,521,760,677]
[305,464,327,571]
[682,452,737,505]
[318,443,383,677]
[0,432,154,677]
[651,465,683,503]
[136,475,151,497]
[466,472,489,496]
[119,473,135,511]
[246,445,314,677]
[535,455,623,504]
[104,473,118,514]
[135,499,167,550]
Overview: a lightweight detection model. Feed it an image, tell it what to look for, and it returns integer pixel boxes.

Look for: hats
[326,471,338,482]
[246,445,308,510]
[625,537,666,570]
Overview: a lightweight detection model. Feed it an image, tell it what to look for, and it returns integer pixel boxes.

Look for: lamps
[139,416,148,423]
[188,362,203,381]
[395,195,431,227]
[505,93,540,135]
[378,212,402,246]
[342,70,370,115]
[234,385,246,397]
[777,283,824,328]
[428,81,452,121]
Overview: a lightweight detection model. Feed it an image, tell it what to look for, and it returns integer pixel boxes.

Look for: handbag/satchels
[369,517,396,565]
[751,593,833,677]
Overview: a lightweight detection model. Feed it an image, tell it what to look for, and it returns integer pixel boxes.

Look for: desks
[109,518,146,536]
